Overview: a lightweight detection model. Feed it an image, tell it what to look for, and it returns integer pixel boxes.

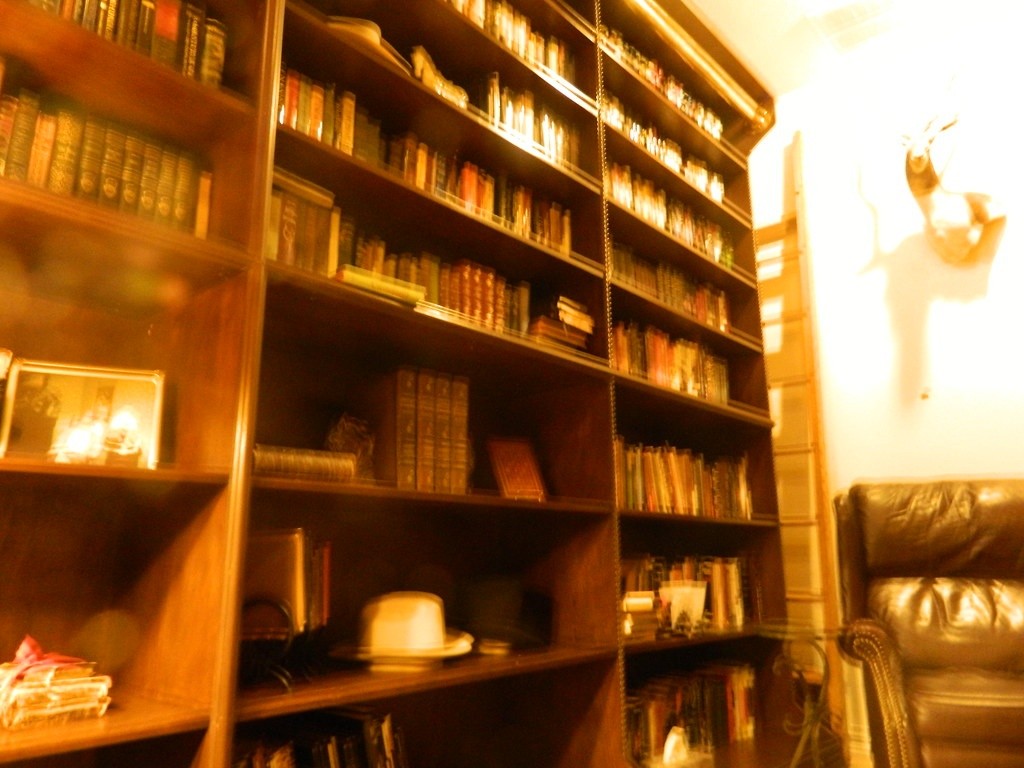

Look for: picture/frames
[0,357,166,471]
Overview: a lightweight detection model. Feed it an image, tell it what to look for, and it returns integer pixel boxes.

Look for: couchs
[833,477,1024,768]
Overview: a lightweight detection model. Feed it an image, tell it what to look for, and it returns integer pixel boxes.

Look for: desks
[661,617,862,768]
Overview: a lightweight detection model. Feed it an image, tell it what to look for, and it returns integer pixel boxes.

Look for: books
[240,0,766,768]
[2,0,232,85]
[2,85,216,243]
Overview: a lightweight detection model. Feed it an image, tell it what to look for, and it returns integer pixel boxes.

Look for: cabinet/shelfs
[1,0,802,768]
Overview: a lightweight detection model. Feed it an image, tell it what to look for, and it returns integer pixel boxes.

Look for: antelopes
[899,114,1006,263]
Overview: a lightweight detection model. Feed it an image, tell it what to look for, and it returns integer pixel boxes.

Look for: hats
[328,591,473,660]
